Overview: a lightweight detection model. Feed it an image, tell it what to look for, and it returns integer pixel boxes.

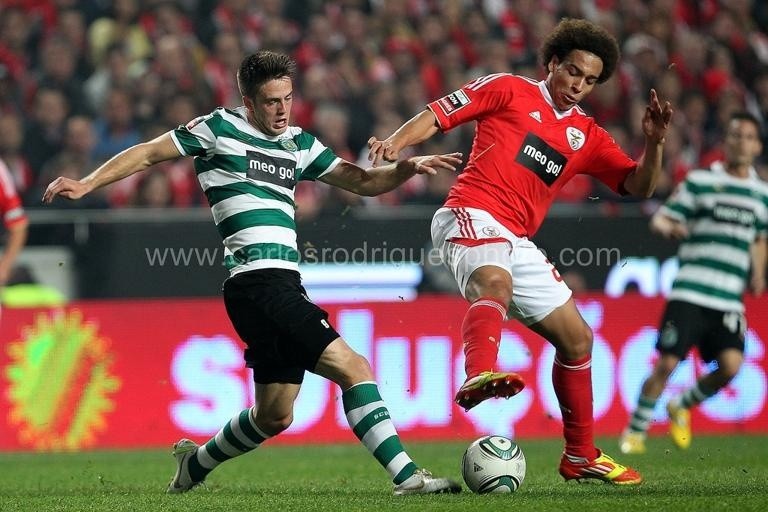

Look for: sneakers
[664,396,693,450]
[558,447,642,487]
[618,423,646,456]
[454,368,527,412]
[165,436,200,497]
[393,468,462,497]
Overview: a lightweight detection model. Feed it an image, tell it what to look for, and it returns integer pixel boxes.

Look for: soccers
[462,436,526,494]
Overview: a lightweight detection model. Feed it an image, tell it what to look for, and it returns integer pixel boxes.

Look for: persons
[0,154,30,287]
[2,0,768,211]
[610,105,768,462]
[37,45,467,499]
[364,15,674,492]
